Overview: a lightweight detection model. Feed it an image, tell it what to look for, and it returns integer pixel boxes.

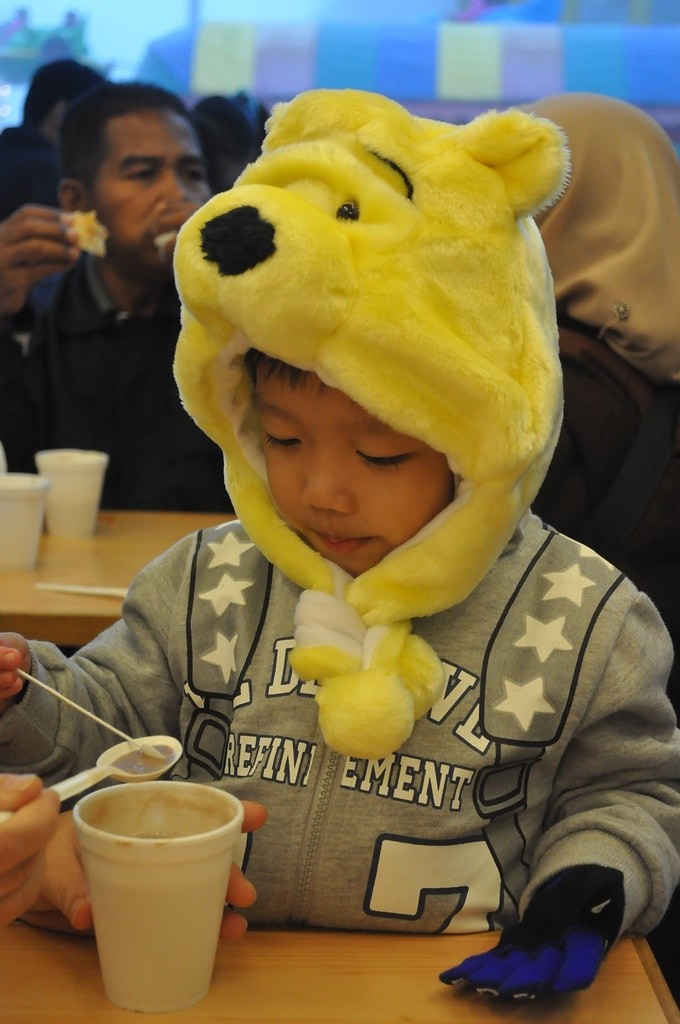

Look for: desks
[0,915,680,1024]
[0,511,235,648]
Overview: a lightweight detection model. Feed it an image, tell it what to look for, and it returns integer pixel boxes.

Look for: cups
[32,449,111,542]
[72,779,247,1015]
[0,471,53,574]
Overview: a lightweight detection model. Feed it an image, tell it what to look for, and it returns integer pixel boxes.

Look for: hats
[174,87,572,760]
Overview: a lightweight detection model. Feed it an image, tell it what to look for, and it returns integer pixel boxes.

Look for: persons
[0,88,680,1003]
[191,95,254,194]
[1,774,270,937]
[0,59,106,222]
[7,81,240,515]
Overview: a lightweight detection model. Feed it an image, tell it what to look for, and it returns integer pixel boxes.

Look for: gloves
[439,864,625,1001]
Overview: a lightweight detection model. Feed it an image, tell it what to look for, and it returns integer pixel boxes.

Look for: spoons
[47,733,183,802]
